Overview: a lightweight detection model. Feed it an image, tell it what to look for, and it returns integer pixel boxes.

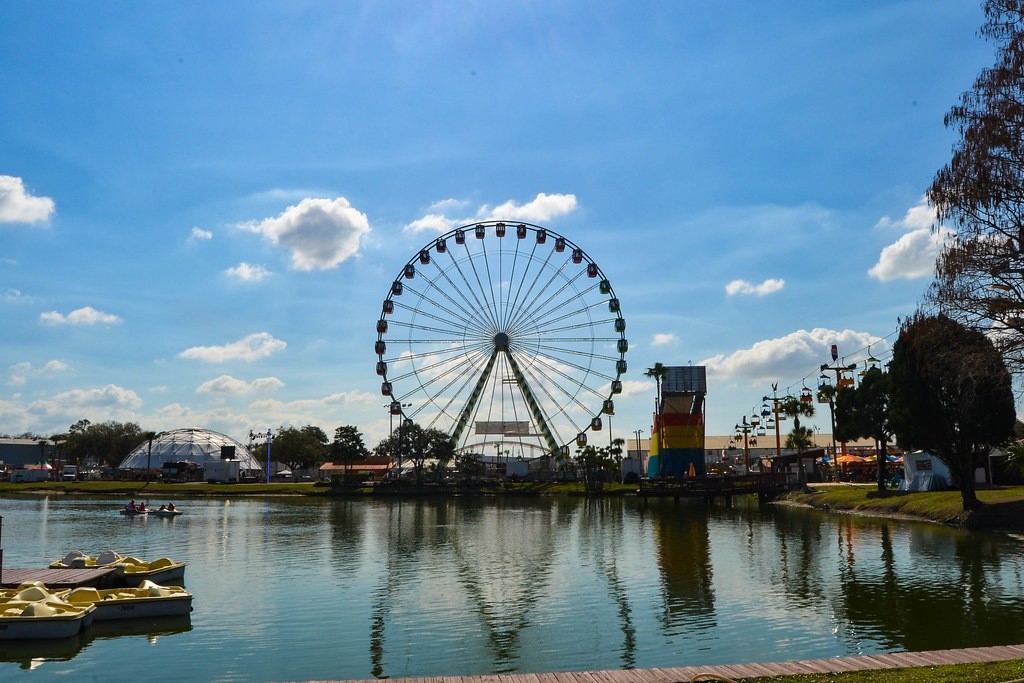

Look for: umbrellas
[819,454,904,473]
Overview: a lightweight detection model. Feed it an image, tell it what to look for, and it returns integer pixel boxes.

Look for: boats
[60,580,194,622]
[0,581,97,641]
[120,508,183,517]
[49,550,187,587]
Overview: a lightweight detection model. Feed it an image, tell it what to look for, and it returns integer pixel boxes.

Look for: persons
[160,502,175,511]
[127,499,146,511]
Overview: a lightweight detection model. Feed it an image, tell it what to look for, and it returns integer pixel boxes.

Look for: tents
[276,470,292,477]
[903,443,986,491]
